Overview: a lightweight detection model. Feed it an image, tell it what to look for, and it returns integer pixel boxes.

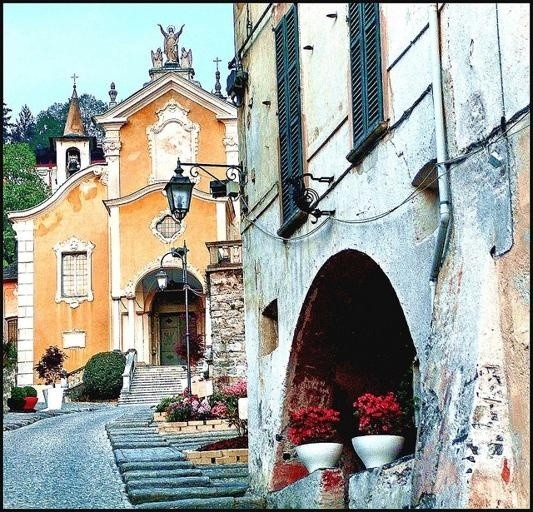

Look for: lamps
[163,156,245,224]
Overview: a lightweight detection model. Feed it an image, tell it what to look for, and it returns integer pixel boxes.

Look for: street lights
[155,239,193,397]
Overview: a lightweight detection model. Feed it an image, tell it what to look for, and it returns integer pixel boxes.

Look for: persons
[179,47,189,69]
[157,24,184,63]
[152,48,162,68]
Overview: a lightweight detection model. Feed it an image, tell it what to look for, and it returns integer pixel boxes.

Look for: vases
[351,434,405,469]
[294,443,344,474]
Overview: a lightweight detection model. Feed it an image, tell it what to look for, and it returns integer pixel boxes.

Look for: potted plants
[32,344,70,410]
[7,385,38,413]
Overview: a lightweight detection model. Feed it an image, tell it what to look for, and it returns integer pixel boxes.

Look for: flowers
[286,403,341,446]
[350,391,407,436]
